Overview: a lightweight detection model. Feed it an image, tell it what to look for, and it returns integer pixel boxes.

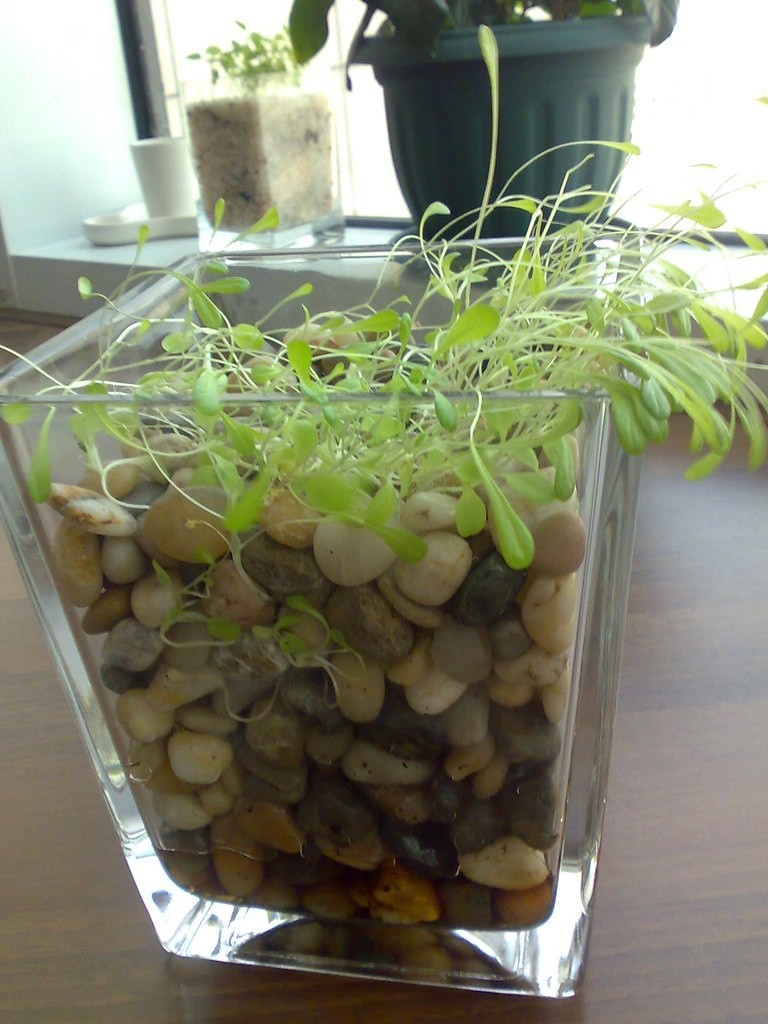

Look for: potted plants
[180,16,346,267]
[0,26,768,1005]
[288,0,682,286]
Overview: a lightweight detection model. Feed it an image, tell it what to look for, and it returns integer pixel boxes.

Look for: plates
[85,205,198,246]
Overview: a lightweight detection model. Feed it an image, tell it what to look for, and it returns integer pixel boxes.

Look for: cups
[130,140,196,220]
